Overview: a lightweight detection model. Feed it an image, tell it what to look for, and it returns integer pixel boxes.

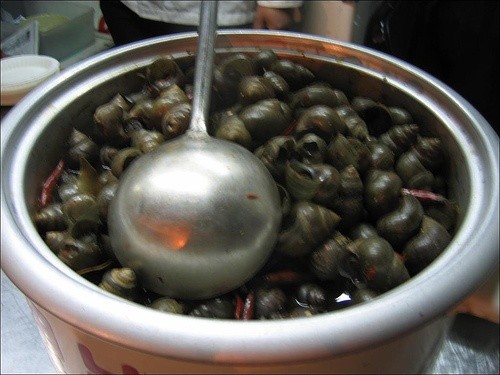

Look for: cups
[1,54,61,120]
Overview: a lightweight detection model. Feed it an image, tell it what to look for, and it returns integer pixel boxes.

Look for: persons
[99,0,304,48]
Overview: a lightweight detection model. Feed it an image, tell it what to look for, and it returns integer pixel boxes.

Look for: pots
[1,29,500,374]
